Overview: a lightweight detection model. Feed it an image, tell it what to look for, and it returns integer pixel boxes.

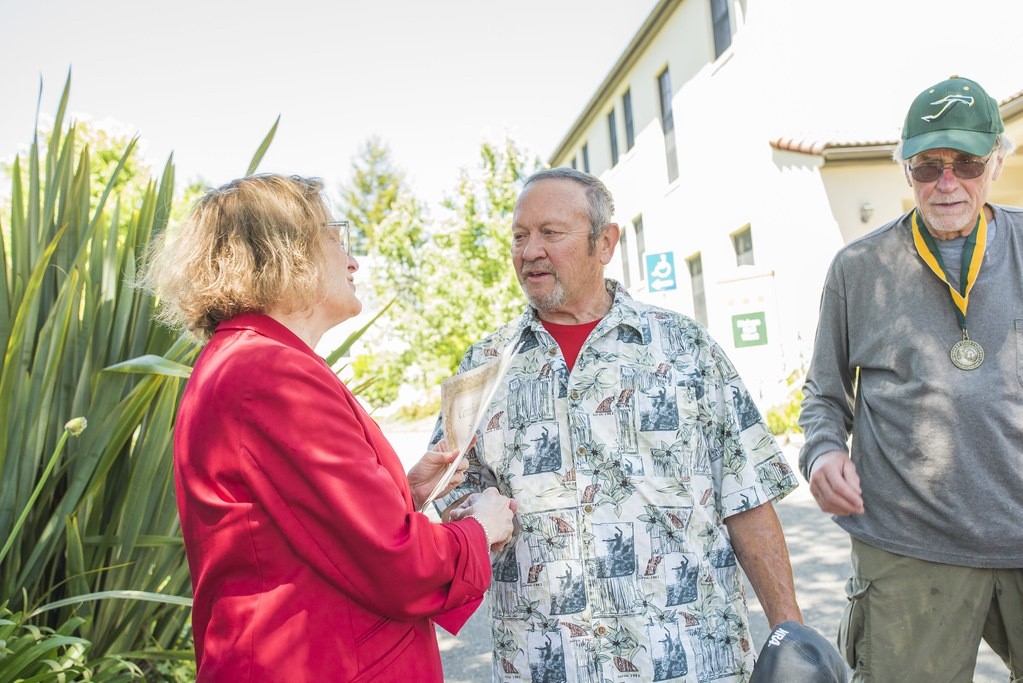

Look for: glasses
[908,149,993,182]
[321,220,351,256]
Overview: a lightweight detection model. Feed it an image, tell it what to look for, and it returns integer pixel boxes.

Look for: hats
[901,74,1004,160]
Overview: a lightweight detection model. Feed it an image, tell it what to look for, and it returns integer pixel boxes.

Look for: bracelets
[462,515,491,553]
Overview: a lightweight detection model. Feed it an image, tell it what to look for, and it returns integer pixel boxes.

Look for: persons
[144,174,513,683]
[427,169,804,683]
[798,75,1023,683]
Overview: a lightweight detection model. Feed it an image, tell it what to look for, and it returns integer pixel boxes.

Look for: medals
[950,340,984,371]
